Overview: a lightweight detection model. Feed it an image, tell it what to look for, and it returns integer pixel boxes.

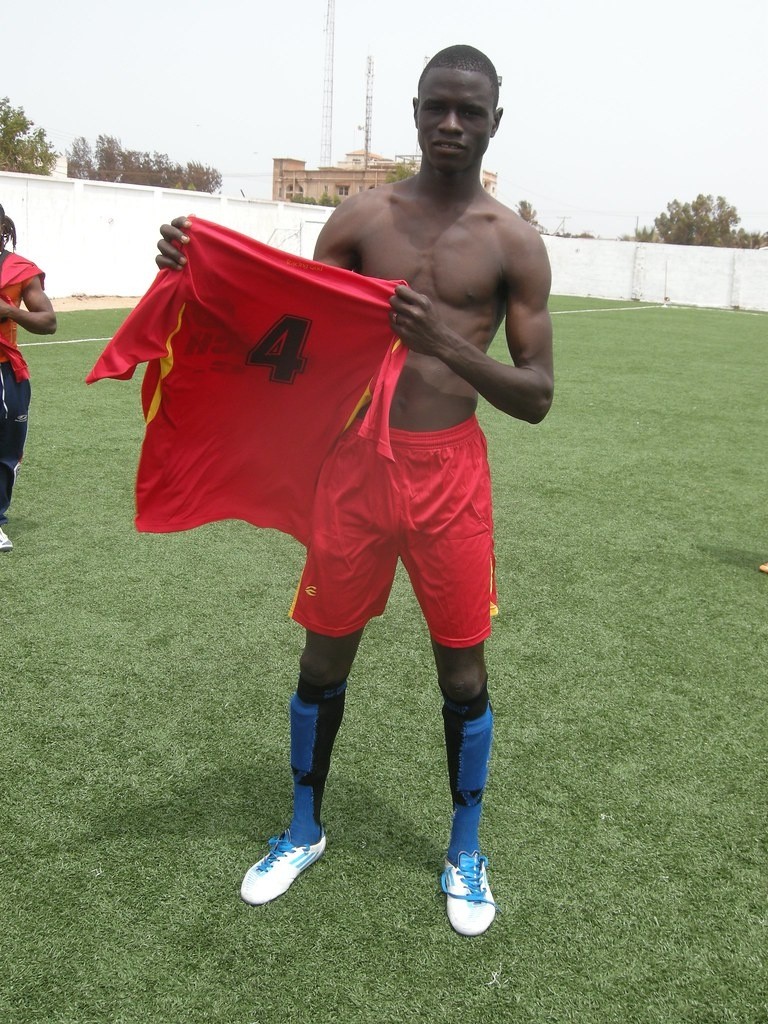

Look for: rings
[392,311,399,324]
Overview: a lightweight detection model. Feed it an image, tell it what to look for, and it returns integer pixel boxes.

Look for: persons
[154,45,555,938]
[0,205,58,554]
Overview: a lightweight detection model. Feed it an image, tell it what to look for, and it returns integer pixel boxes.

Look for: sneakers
[241,824,326,905]
[0,527,13,551]
[441,850,502,936]
